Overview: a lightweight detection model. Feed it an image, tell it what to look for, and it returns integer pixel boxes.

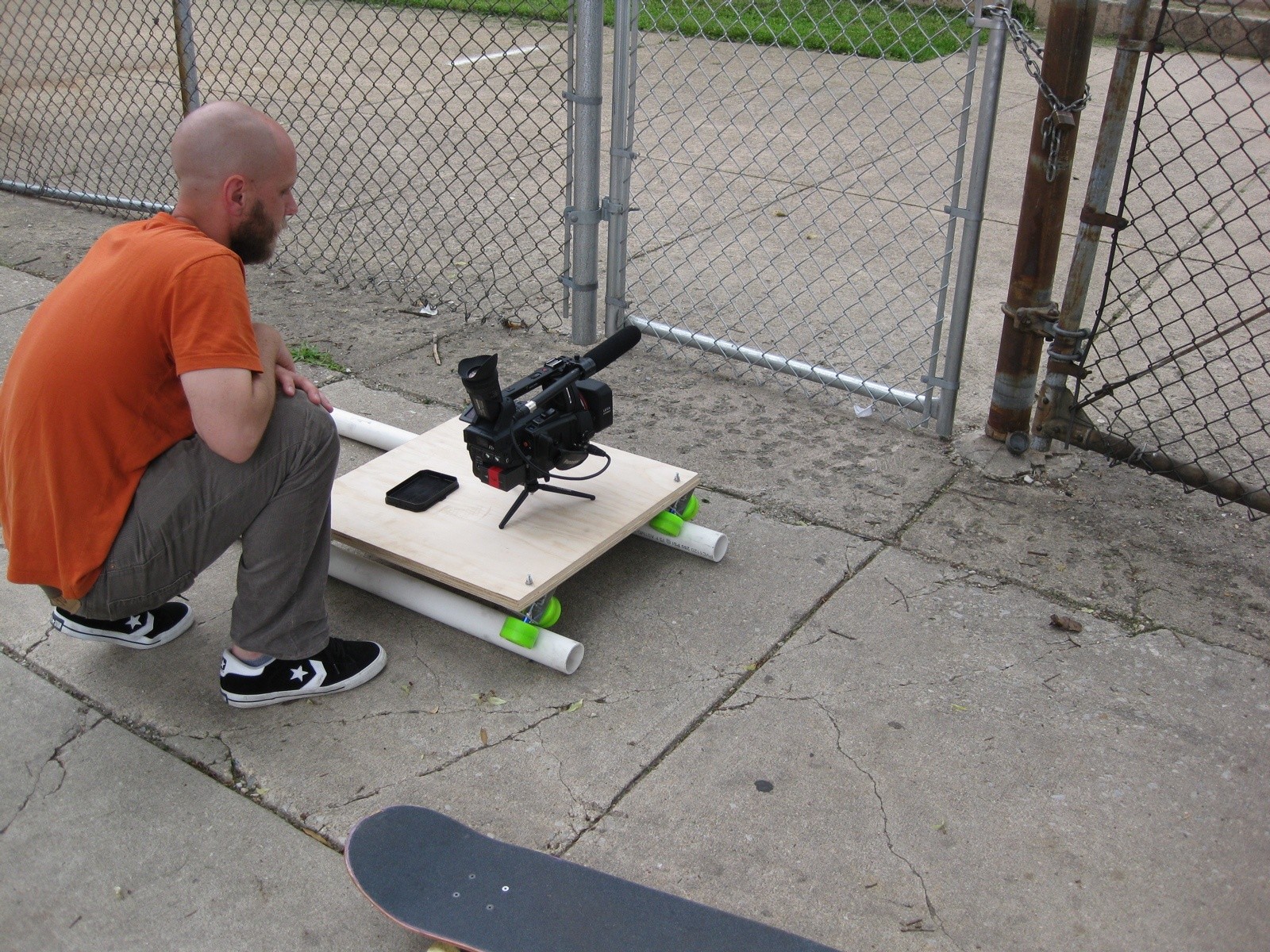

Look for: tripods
[498,477,595,530]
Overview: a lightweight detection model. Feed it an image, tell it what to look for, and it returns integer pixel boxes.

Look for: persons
[0,102,386,709]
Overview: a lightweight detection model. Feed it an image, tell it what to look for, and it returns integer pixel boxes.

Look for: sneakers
[50,593,195,649]
[219,635,387,708]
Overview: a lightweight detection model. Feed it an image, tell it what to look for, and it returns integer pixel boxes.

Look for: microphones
[516,326,642,419]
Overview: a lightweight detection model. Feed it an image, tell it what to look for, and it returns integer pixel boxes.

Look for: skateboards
[343,807,850,952]
